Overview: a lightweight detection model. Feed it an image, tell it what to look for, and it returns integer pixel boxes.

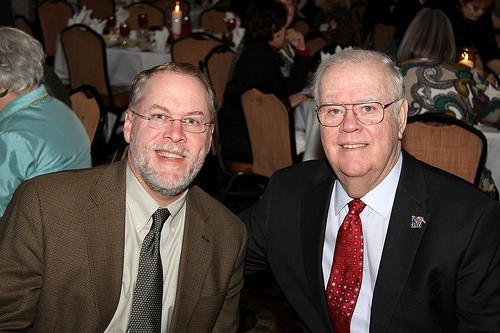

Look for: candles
[171,4,182,35]
[459,54,475,69]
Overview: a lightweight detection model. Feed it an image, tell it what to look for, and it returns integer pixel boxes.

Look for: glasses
[129,108,213,134]
[316,98,400,127]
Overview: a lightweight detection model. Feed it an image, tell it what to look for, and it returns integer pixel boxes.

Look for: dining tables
[293,88,324,162]
[473,122,500,199]
[53,24,203,92]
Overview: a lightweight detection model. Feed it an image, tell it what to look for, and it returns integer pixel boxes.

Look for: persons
[0,61,248,333]
[217,0,500,333]
[0,27,92,219]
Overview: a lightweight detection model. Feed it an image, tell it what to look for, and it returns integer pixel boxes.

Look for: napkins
[64,5,108,36]
[484,83,500,100]
[154,27,170,51]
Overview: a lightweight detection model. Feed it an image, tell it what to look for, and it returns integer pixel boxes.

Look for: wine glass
[120,22,130,49]
[107,16,116,35]
[138,14,148,38]
[223,15,237,43]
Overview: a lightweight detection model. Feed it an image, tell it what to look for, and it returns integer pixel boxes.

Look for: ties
[126,208,172,333]
[326,199,367,333]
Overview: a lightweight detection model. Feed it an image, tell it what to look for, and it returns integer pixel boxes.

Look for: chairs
[0,0,488,187]
